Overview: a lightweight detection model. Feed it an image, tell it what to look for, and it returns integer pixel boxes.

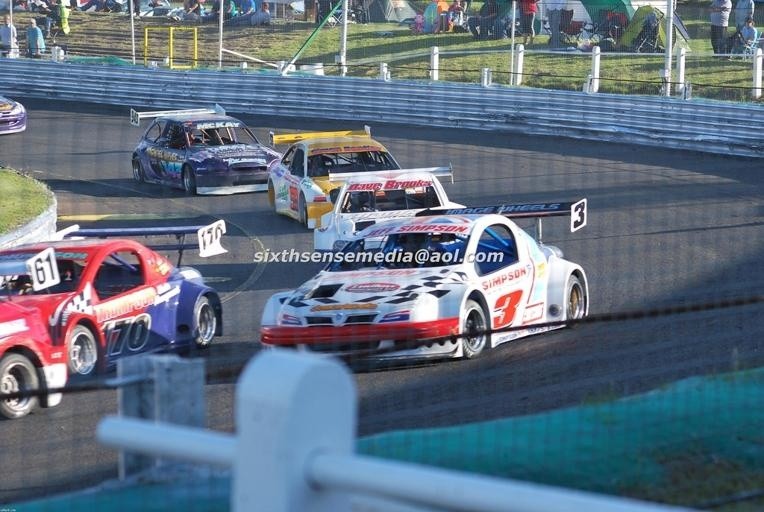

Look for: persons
[45,0,71,44]
[468,0,498,39]
[434,0,464,33]
[11,0,271,28]
[0,14,17,58]
[705,1,758,61]
[25,18,46,59]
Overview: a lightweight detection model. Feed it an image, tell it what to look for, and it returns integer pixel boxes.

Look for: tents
[481,0,692,54]
[423,0,450,33]
[365,0,418,23]
